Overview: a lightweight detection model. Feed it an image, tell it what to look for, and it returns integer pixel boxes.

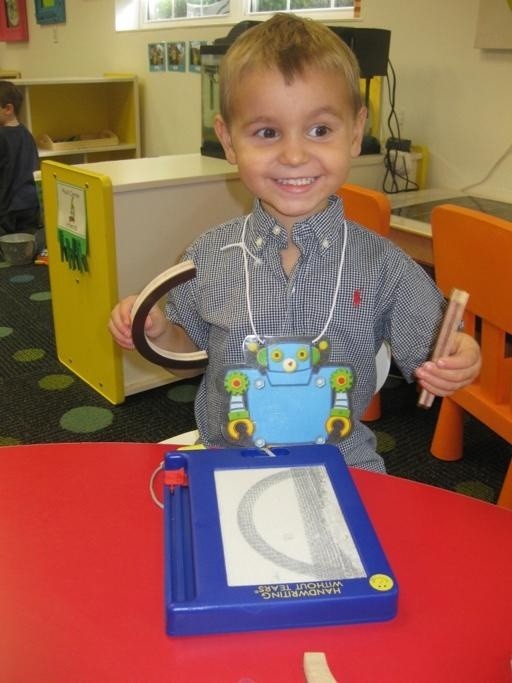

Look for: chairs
[334,184,391,420]
[430,204,512,506]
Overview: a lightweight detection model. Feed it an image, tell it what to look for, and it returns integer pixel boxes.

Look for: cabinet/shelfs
[0,71,140,163]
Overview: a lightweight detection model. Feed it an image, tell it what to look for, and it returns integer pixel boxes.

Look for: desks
[1,442,512,683]
[383,188,510,267]
[41,150,424,405]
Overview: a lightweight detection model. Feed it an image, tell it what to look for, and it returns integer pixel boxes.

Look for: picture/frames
[0,0,28,41]
[35,0,66,24]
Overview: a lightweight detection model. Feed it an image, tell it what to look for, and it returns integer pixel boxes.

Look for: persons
[1,80,51,265]
[106,13,482,480]
